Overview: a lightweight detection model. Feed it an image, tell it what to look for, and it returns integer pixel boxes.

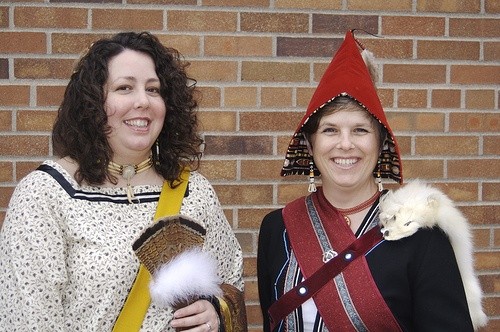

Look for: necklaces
[98,158,154,201]
[336,191,381,226]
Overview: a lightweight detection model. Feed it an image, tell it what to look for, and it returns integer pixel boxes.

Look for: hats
[280,30,403,185]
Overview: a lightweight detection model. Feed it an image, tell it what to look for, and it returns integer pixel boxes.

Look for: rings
[207,324,211,330]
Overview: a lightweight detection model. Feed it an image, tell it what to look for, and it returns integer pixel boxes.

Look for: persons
[255,30,474,332]
[0,32,244,332]
